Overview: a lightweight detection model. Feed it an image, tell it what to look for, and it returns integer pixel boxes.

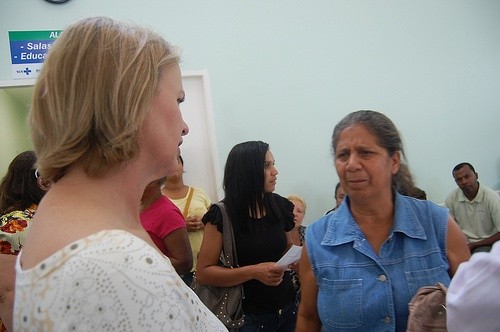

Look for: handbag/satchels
[189,202,247,329]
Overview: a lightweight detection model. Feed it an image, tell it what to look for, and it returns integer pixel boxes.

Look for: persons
[162,155,213,286]
[13,16,229,332]
[444,163,500,254]
[196,140,302,332]
[0,150,52,332]
[139,176,194,276]
[287,110,472,332]
